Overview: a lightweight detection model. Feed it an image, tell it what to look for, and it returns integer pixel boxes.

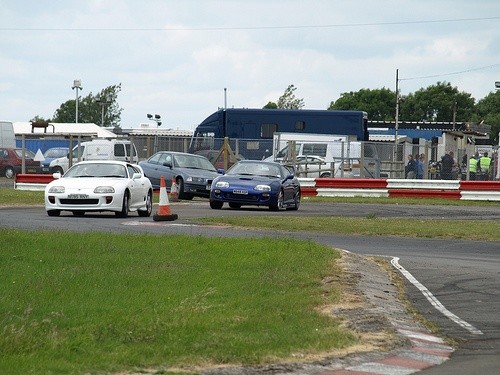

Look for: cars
[210,159,301,212]
[44,160,153,218]
[284,154,331,178]
[0,146,70,179]
[138,151,221,201]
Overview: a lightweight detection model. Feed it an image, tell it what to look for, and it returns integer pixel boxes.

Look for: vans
[257,139,381,178]
[49,138,139,176]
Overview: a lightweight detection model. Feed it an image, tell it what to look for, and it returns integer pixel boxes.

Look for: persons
[406,154,424,179]
[469,152,498,181]
[442,152,454,179]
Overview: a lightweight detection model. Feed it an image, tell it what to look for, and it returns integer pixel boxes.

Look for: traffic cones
[168,175,181,202]
[153,175,178,221]
[72,79,83,123]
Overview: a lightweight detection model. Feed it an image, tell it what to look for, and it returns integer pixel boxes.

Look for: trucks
[186,108,369,160]
